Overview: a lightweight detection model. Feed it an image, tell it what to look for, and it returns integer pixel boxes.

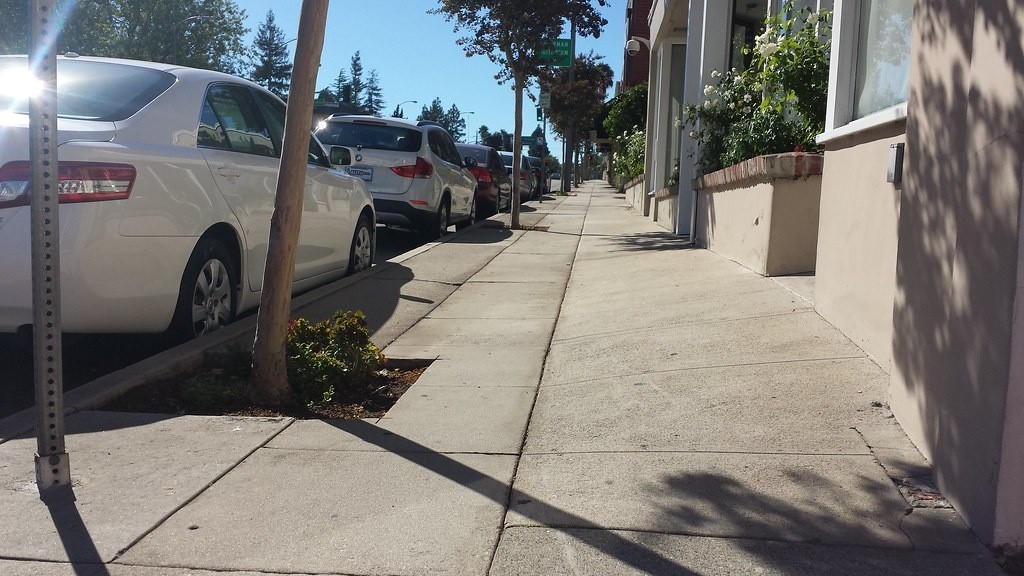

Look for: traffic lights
[588,156,591,160]
[545,160,548,166]
[590,148,592,152]
[537,108,542,121]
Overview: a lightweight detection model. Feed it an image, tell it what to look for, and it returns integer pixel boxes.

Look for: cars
[550,173,560,180]
[456,143,512,218]
[0,52,376,350]
[496,151,552,201]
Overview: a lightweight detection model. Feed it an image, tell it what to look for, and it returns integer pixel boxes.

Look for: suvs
[313,115,478,240]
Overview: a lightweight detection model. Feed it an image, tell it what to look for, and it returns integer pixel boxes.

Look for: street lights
[395,100,417,116]
